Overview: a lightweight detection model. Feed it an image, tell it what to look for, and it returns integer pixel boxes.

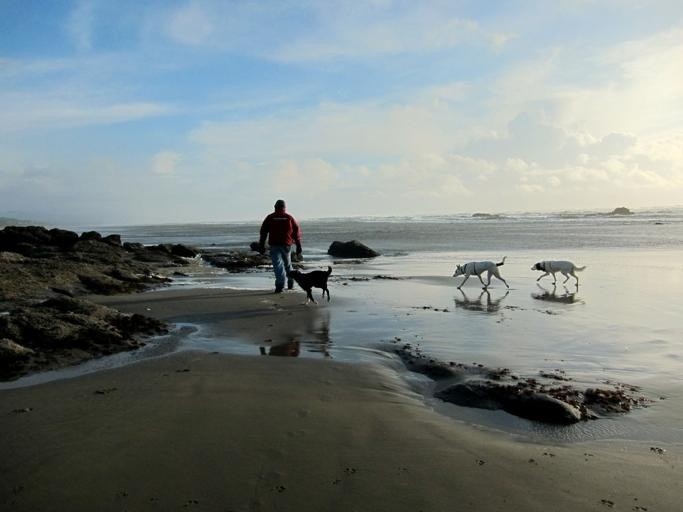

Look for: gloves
[259,240,266,253]
[296,242,302,254]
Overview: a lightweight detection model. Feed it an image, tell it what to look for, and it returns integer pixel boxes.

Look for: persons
[260,200,302,292]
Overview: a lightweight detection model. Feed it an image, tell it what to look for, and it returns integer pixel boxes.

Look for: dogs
[453,256,509,289]
[531,261,586,286]
[286,266,332,305]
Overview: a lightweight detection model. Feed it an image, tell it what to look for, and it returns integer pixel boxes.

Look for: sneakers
[275,287,283,293]
[288,279,293,289]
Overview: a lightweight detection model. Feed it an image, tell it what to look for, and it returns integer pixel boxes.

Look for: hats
[275,200,286,206]
